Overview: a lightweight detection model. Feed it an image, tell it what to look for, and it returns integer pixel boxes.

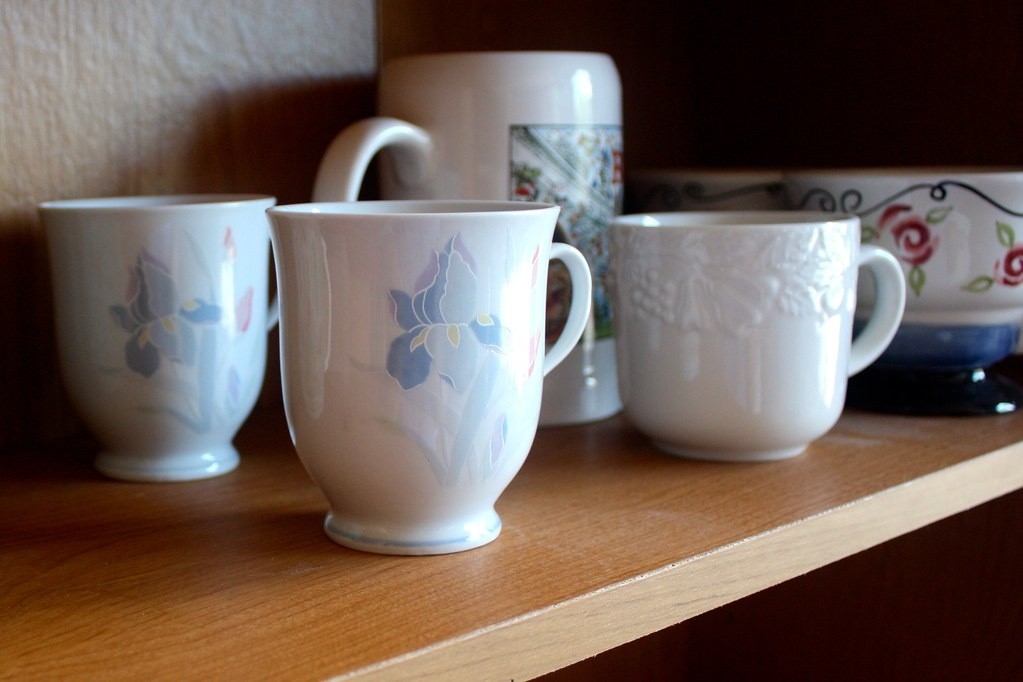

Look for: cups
[317,53,624,426]
[265,201,592,555]
[667,171,1023,379]
[39,195,279,482]
[612,211,906,460]
